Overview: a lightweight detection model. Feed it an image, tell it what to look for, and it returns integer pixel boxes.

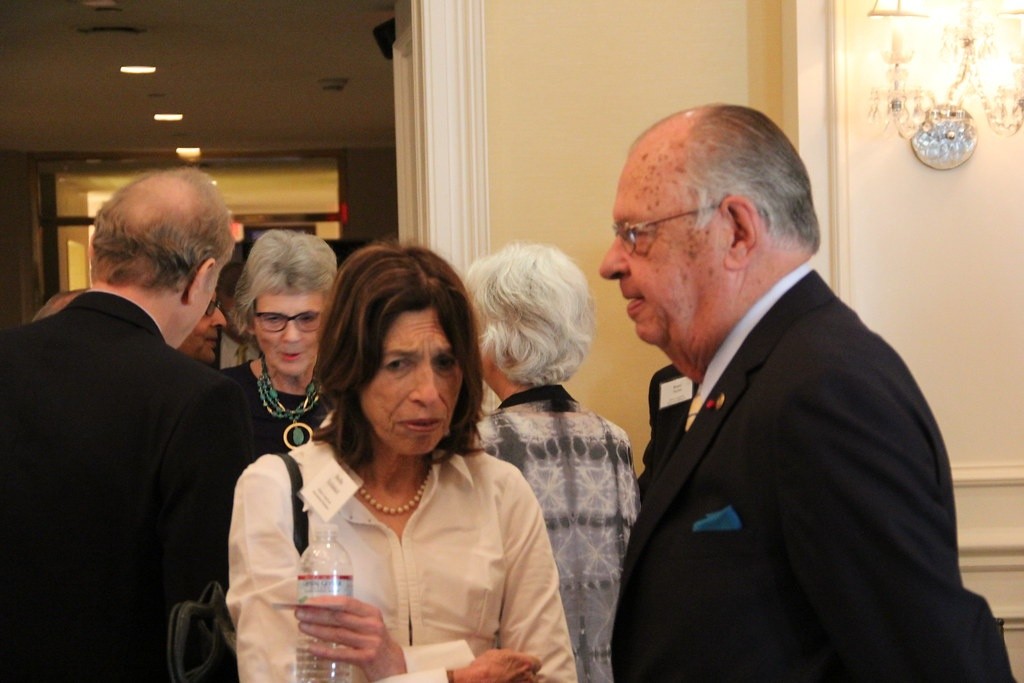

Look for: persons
[228,246,576,683]
[0,105,1016,683]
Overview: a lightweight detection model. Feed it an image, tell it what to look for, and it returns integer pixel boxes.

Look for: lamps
[865,0,1024,138]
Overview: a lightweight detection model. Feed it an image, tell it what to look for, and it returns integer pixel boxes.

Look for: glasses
[205,300,221,316]
[611,205,720,254]
[253,303,321,332]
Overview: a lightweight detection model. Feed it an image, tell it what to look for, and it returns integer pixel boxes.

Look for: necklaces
[257,353,321,448]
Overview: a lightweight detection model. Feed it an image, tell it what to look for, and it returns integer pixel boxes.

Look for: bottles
[296,521,355,683]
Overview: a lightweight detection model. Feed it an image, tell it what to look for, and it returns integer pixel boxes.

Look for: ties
[235,345,247,365]
[684,394,703,432]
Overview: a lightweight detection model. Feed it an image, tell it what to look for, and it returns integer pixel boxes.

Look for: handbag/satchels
[166,451,308,683]
